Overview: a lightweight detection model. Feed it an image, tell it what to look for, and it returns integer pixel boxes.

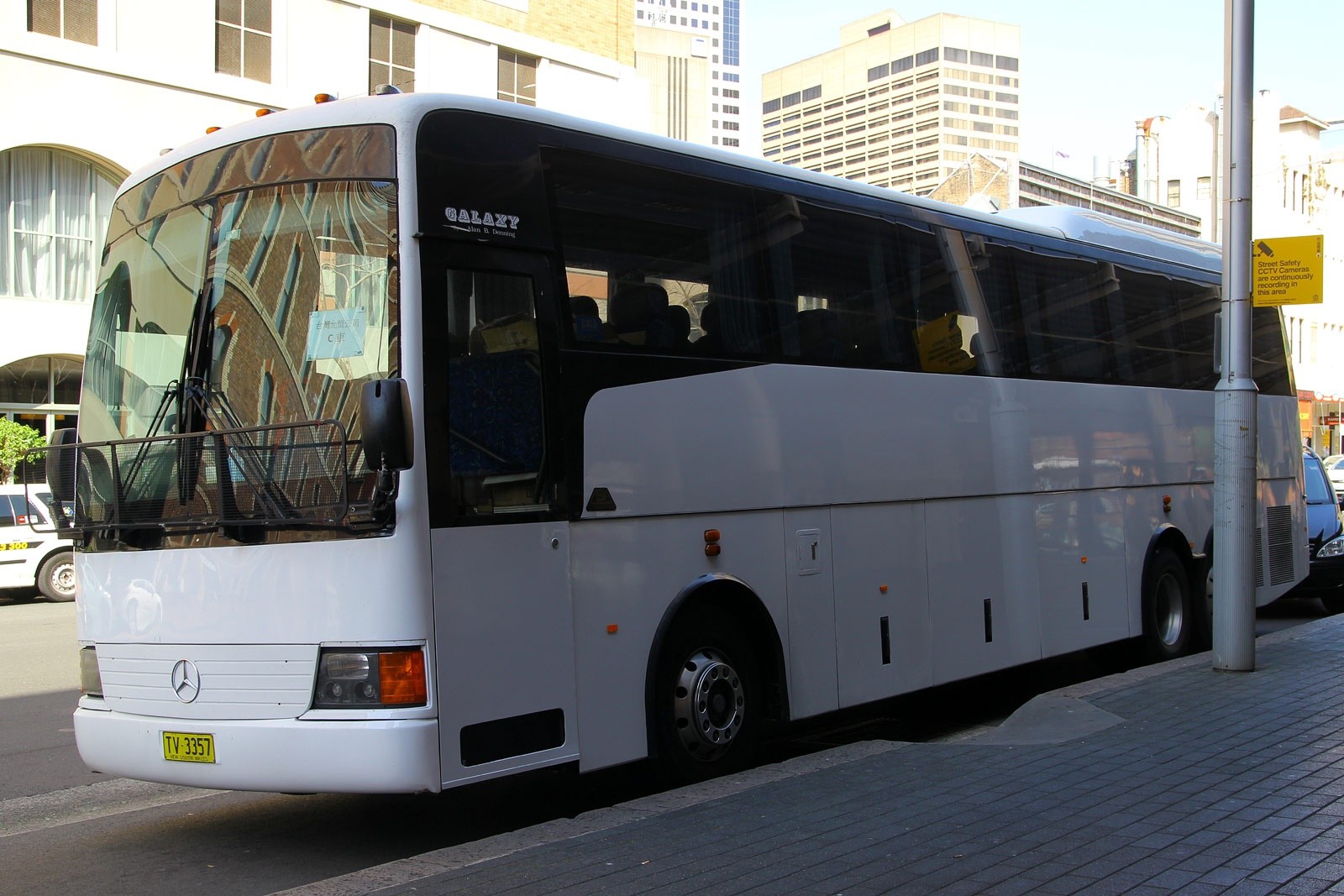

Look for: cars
[1322,454,1344,504]
[1300,442,1344,610]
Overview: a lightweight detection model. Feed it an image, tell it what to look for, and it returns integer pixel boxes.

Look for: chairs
[570,284,986,373]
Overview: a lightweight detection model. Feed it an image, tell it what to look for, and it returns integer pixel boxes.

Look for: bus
[21,88,1309,791]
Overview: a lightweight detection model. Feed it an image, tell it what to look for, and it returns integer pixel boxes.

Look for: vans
[0,485,76,602]
